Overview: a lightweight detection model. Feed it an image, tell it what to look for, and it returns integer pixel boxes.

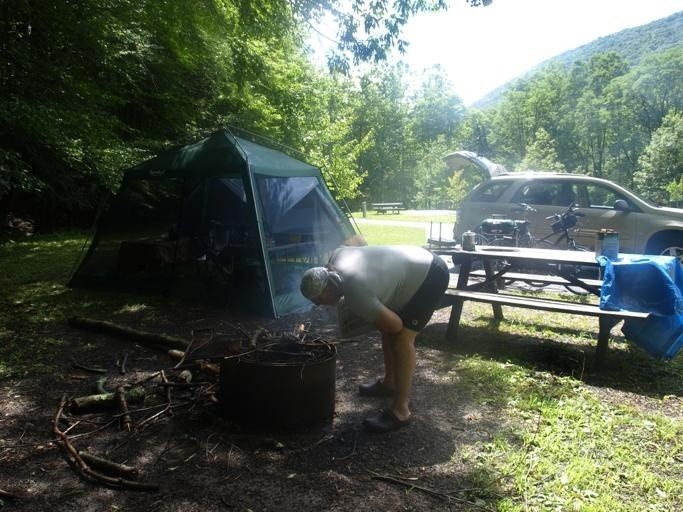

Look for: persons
[300,233,450,434]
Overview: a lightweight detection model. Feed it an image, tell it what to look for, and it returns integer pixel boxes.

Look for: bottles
[462,230,476,251]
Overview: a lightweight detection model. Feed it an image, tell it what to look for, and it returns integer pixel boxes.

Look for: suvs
[441,150,683,269]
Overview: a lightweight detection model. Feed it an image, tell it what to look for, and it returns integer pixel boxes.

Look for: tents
[64,120,364,318]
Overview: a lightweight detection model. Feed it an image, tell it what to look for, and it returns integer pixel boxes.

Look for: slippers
[359,378,394,396]
[363,409,410,432]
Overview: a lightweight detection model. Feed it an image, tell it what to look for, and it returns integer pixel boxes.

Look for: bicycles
[451,201,603,295]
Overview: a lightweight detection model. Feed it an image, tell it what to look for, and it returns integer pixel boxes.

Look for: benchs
[468,267,606,320]
[442,288,652,371]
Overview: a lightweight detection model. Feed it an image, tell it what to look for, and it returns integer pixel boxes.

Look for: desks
[429,244,614,371]
